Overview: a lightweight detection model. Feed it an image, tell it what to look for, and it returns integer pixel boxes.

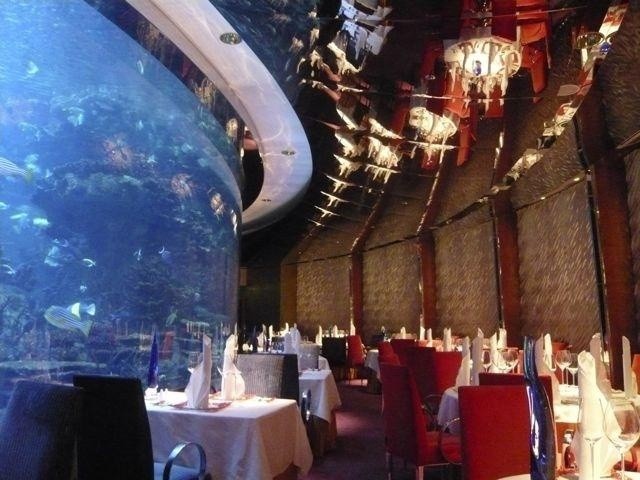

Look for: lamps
[444,0,524,114]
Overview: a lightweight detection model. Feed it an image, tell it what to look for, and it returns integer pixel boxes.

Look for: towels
[269,325,273,338]
[420,325,453,351]
[319,321,356,338]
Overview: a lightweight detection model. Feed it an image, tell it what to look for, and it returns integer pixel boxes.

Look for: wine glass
[576,397,640,480]
[544,349,579,392]
[480,348,519,373]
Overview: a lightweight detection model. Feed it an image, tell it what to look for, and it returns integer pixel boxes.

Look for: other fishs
[0,57,219,338]
